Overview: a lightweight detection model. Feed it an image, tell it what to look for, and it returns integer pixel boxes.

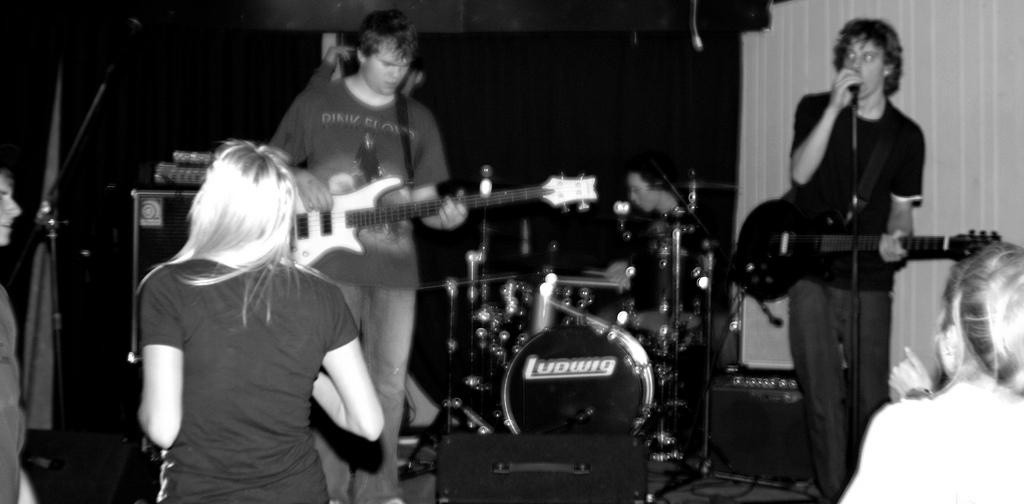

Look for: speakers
[435,432,646,504]
[705,380,813,489]
[128,190,199,363]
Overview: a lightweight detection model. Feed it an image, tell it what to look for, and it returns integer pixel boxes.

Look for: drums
[538,275,626,327]
[631,307,694,358]
[502,319,663,438]
[482,280,535,322]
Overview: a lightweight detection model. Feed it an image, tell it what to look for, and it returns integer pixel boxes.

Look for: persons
[827,238,1024,504]
[0,150,42,504]
[129,130,386,504]
[783,17,925,503]
[600,148,736,393]
[261,4,470,504]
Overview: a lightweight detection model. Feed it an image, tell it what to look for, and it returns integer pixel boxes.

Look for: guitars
[731,200,1004,304]
[283,175,600,271]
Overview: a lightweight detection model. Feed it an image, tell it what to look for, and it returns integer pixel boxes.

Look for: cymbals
[647,172,737,193]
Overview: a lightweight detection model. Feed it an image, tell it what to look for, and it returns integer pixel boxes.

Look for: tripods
[645,225,790,504]
[397,279,499,482]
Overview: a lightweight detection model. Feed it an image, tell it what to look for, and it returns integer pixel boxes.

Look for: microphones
[848,82,861,106]
[109,16,142,72]
